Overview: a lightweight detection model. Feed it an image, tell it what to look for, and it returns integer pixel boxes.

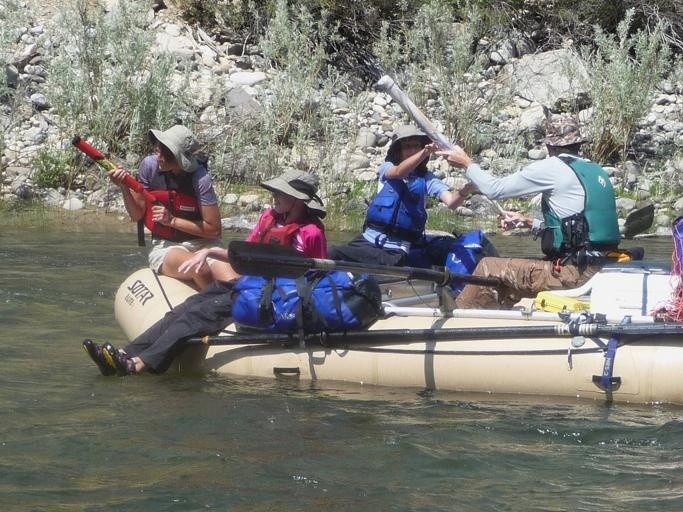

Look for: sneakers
[83,339,116,376]
[102,343,135,376]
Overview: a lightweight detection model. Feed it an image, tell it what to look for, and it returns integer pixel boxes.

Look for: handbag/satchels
[409,230,499,287]
[230,270,381,333]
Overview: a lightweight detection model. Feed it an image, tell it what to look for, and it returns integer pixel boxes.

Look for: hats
[261,169,327,219]
[147,125,201,172]
[536,119,588,147]
[387,126,432,155]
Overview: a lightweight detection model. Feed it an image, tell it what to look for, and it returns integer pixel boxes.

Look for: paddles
[185,316,683,343]
[228,241,503,287]
[617,206,653,239]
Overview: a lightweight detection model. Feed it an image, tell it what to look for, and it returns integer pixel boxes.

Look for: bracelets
[459,190,467,198]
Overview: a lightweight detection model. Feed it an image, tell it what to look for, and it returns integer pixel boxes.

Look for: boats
[113,259,683,410]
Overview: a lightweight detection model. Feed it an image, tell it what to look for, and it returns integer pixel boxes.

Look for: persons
[325,124,477,273]
[435,117,620,309]
[82,169,328,376]
[108,125,243,290]
